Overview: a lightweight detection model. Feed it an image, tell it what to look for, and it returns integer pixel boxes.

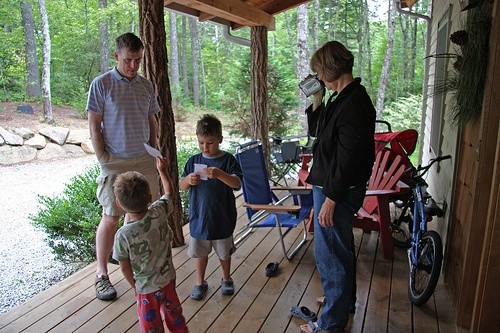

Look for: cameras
[299,75,325,97]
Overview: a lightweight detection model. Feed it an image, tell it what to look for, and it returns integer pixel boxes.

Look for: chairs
[233,138,411,260]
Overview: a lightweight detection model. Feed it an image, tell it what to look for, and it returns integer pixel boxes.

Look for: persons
[85,32,163,300]
[110,156,188,333]
[300,40,376,333]
[178,113,244,300]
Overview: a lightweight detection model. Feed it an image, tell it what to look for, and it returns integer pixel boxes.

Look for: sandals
[318,295,356,313]
[300,320,341,333]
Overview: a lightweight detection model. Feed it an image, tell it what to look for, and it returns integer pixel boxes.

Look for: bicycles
[388,155,453,307]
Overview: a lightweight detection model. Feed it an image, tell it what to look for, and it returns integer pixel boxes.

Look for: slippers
[221,277,234,295]
[190,280,209,300]
[290,305,318,322]
[265,261,279,277]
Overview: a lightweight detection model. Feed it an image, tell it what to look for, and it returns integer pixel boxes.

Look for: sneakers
[94,273,118,300]
[107,250,119,264]
